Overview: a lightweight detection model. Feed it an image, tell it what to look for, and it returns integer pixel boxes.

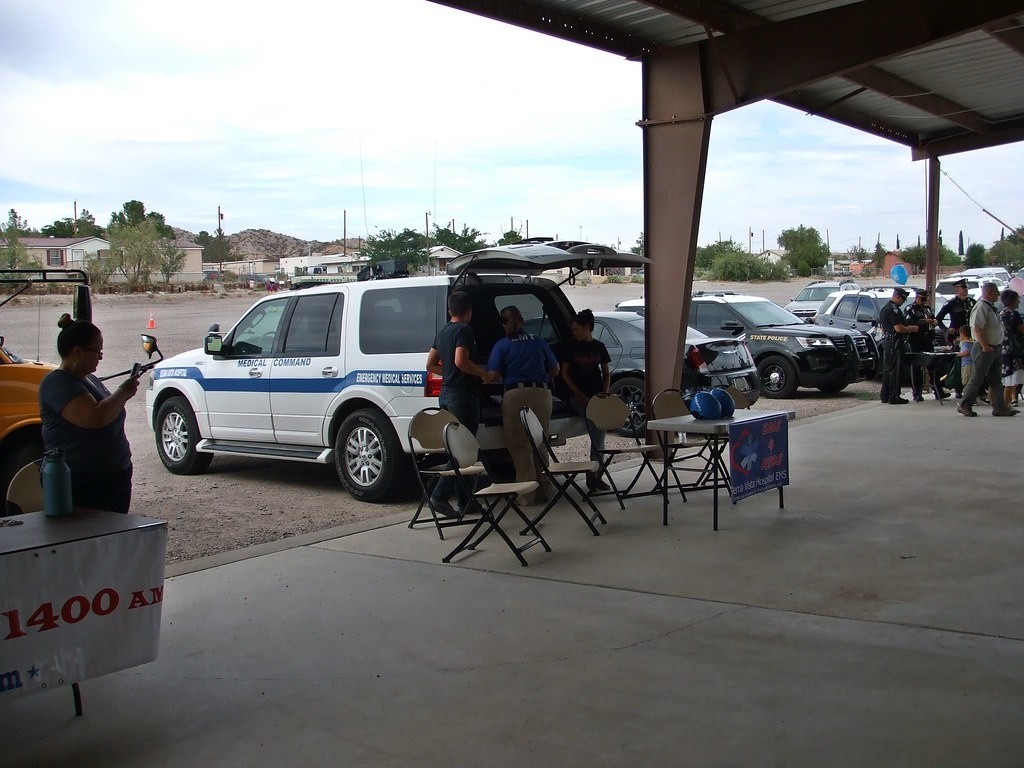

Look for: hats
[914,288,929,300]
[952,278,969,288]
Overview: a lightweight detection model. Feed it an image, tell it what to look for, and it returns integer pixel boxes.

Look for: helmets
[710,388,735,419]
[690,391,722,420]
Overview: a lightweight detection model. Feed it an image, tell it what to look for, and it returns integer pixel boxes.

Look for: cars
[936,265,1024,318]
[515,311,762,437]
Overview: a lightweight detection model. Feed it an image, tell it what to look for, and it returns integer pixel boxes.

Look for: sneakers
[957,400,977,406]
[534,484,552,502]
[956,394,962,398]
[458,501,482,514]
[956,404,977,417]
[979,397,990,406]
[426,498,462,517]
[513,493,535,506]
[992,408,1020,417]
[1010,400,1018,407]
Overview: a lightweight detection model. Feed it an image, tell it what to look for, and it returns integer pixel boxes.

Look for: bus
[0,267,166,518]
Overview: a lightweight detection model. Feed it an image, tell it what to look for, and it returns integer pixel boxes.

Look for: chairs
[306,305,339,353]
[519,405,607,536]
[702,384,750,486]
[582,392,669,511]
[441,420,552,567]
[650,388,731,503]
[373,307,402,352]
[6,459,45,514]
[408,407,495,541]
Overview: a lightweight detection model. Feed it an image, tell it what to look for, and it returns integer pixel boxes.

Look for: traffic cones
[146,310,157,329]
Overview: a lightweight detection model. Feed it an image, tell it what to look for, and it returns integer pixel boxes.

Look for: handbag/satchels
[1008,315,1024,355]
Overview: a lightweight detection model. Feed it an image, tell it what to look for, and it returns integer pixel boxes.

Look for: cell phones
[130,363,142,378]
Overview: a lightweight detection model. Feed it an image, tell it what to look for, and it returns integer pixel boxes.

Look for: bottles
[677,431,688,444]
[42,447,74,516]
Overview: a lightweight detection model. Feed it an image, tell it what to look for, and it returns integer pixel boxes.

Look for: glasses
[894,287,910,301]
[72,344,103,359]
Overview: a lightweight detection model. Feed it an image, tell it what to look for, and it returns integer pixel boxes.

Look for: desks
[906,352,961,406]
[0,508,168,715]
[647,409,796,531]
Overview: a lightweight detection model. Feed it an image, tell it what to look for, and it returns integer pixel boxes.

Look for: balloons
[891,265,909,284]
[1009,277,1024,296]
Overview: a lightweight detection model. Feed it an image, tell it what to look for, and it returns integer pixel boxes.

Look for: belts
[502,381,551,395]
[976,341,1000,348]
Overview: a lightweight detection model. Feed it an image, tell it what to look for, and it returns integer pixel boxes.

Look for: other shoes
[913,396,924,401]
[881,398,909,405]
[935,393,951,399]
[586,472,610,491]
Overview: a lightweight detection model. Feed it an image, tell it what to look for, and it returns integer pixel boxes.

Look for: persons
[487,306,560,505]
[880,287,918,404]
[561,309,612,490]
[935,278,1024,417]
[426,291,492,519]
[902,289,951,401]
[38,313,140,515]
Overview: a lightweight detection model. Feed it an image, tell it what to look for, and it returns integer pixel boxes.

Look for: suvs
[145,235,657,504]
[781,278,863,323]
[612,291,881,400]
[806,285,950,384]
